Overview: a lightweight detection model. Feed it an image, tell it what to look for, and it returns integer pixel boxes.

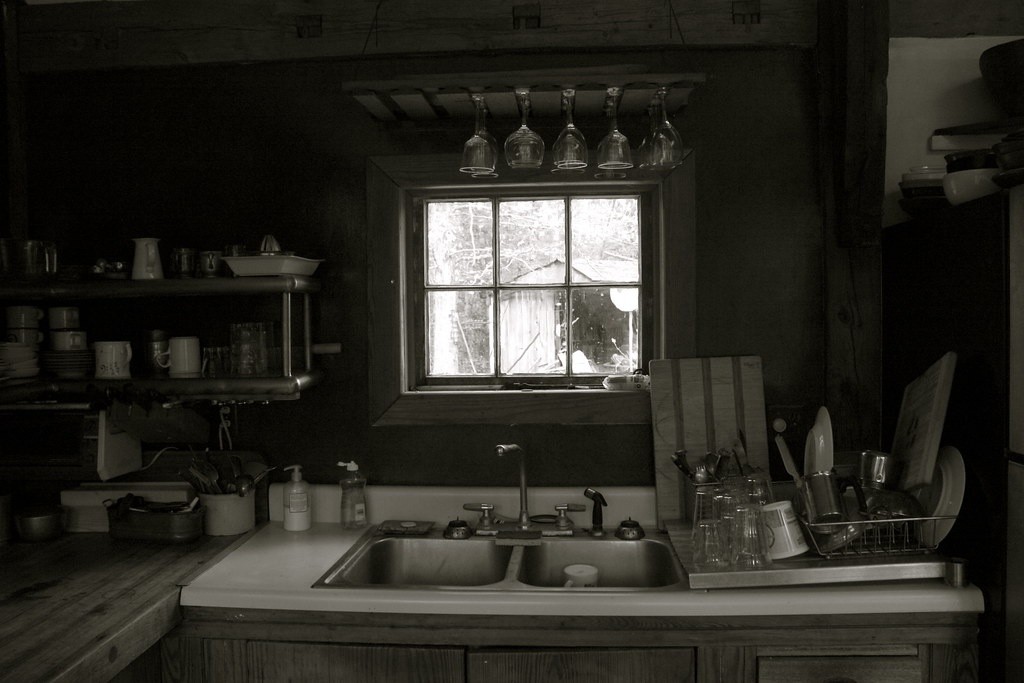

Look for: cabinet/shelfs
[0,274,327,403]
[178,604,719,683]
[743,611,980,683]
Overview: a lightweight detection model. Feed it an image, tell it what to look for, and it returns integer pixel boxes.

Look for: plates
[920,446,966,546]
[803,405,834,474]
[222,255,326,277]
[0,343,94,379]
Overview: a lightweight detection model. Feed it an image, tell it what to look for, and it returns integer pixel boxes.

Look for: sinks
[517,533,689,592]
[309,526,515,592]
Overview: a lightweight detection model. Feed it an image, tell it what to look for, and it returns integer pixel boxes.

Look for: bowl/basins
[15,505,69,540]
[0,238,57,276]
[102,498,200,541]
[898,127,1024,217]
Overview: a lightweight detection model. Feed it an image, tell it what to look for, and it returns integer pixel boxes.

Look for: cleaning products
[283,465,311,531]
[336,461,369,531]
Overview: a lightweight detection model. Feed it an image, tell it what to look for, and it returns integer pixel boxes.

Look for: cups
[156,336,202,378]
[202,251,222,277]
[6,305,86,351]
[200,492,256,536]
[202,322,286,378]
[687,483,771,568]
[130,238,162,280]
[763,500,809,559]
[563,563,598,588]
[166,248,196,279]
[94,341,132,379]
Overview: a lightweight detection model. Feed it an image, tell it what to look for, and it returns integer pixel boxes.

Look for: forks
[190,452,235,492]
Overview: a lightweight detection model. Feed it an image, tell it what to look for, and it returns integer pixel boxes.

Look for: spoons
[235,473,254,495]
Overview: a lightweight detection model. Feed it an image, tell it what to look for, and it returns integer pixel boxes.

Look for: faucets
[462,443,587,537]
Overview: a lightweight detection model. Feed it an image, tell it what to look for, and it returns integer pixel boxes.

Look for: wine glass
[456,82,686,180]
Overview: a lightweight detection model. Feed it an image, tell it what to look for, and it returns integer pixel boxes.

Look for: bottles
[796,474,849,534]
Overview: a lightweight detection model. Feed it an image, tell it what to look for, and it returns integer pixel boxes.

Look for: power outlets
[213,403,239,442]
